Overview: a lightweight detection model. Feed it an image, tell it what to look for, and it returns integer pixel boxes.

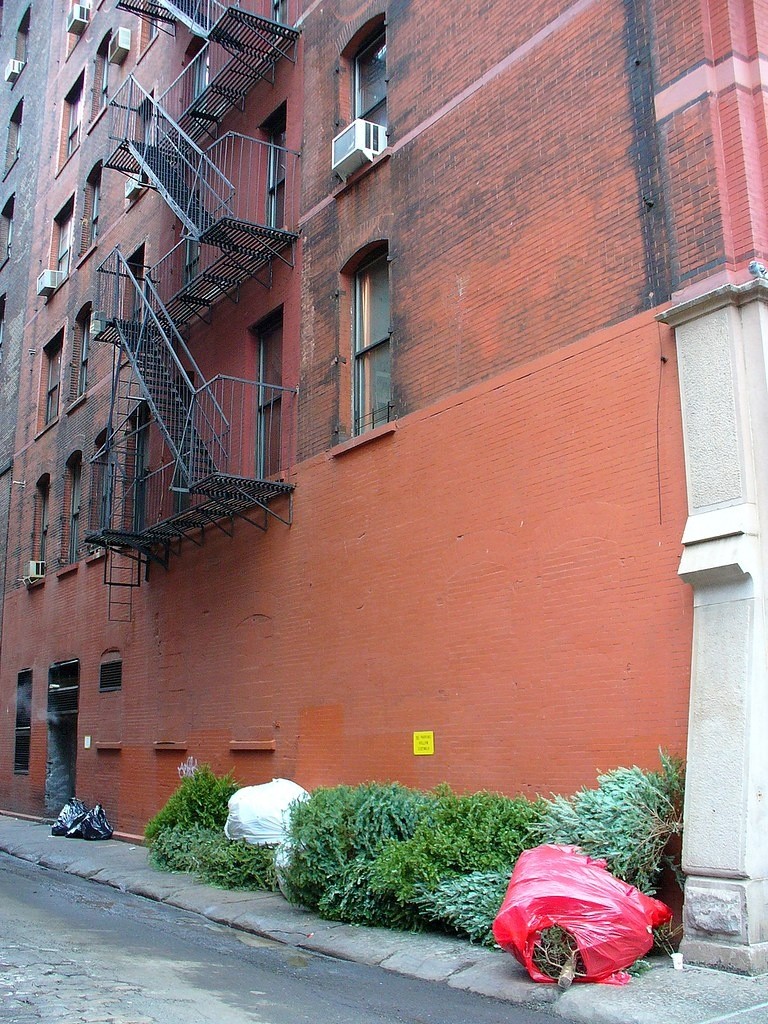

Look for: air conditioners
[22,561,46,587]
[66,4,91,36]
[89,311,107,334]
[107,26,132,64]
[331,119,388,185]
[4,59,26,83]
[36,269,64,297]
[125,174,142,199]
